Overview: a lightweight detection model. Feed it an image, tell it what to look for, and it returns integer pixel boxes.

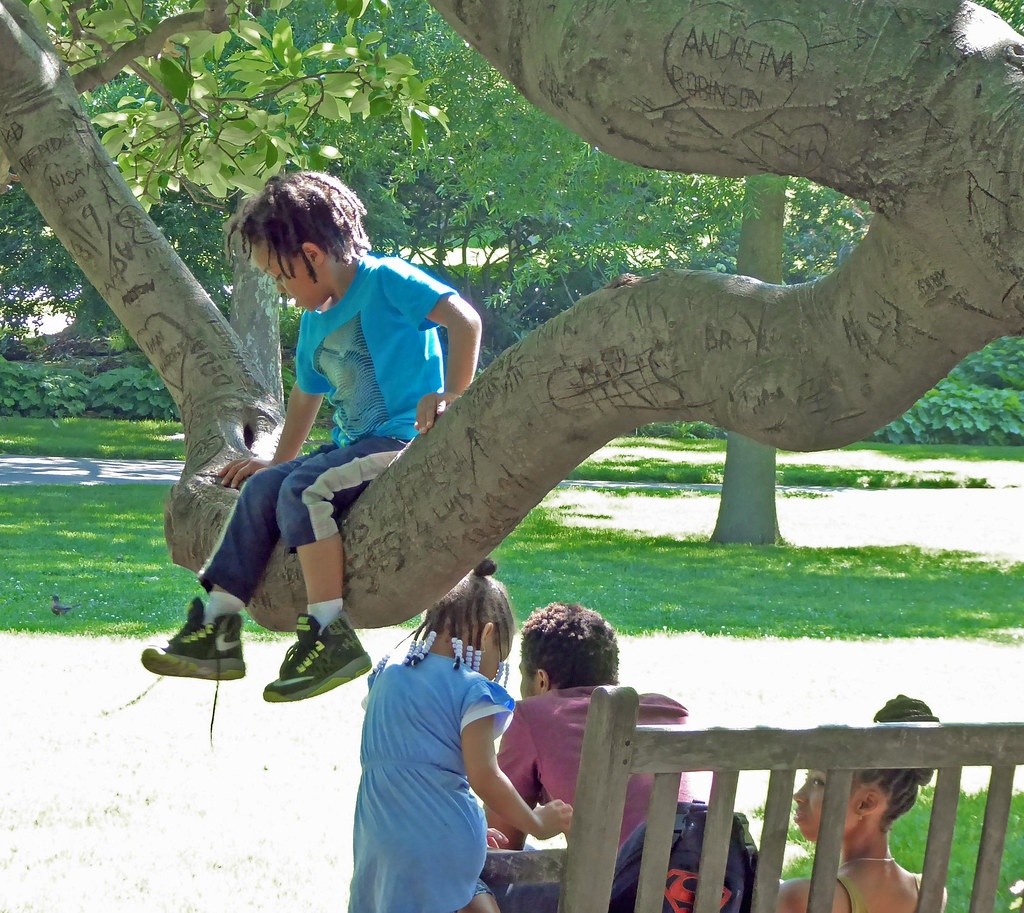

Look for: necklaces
[841,858,894,867]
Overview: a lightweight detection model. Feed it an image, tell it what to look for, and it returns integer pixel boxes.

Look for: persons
[345,557,573,913]
[776,697,949,912]
[484,601,753,913]
[141,172,482,703]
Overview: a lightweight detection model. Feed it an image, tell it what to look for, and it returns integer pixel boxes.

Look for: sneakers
[263,609,373,703]
[140,597,247,743]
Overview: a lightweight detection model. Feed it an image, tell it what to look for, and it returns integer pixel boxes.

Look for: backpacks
[609,799,758,913]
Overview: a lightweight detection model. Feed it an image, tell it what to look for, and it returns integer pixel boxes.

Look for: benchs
[478,685,1024,913]
